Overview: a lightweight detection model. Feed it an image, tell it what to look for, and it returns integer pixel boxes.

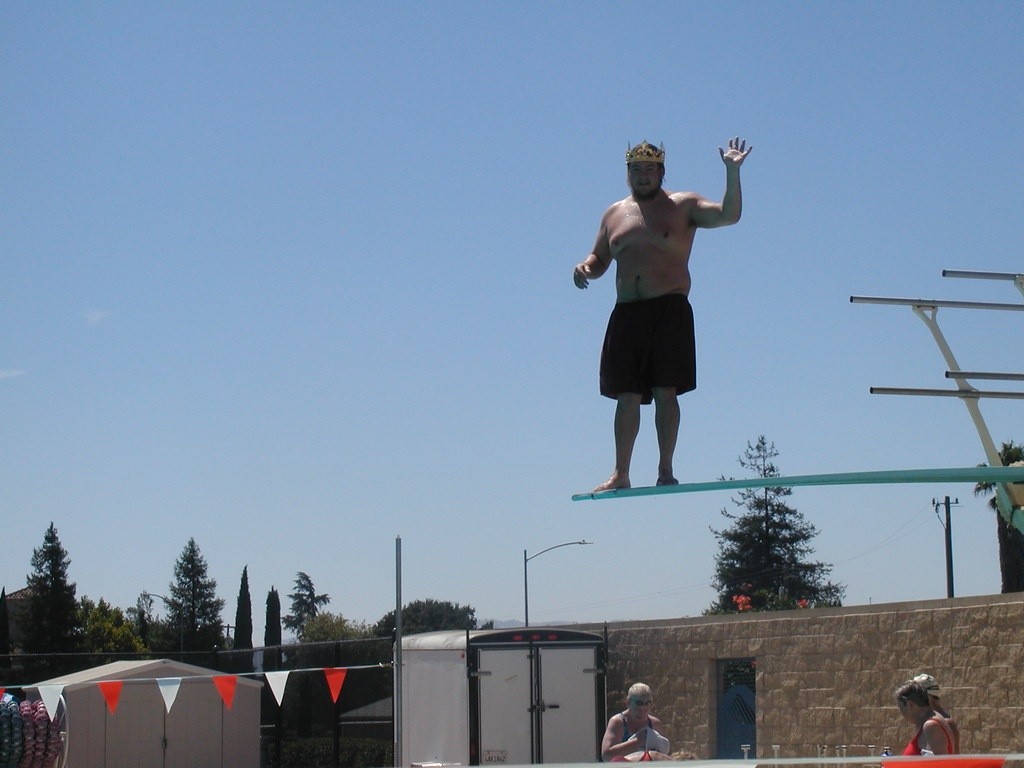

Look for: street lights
[145,594,184,663]
[524,540,594,628]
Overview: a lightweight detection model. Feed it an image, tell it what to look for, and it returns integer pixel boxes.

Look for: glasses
[630,699,653,707]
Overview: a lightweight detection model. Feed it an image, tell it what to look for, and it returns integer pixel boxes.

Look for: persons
[573,136,753,492]
[896,681,956,755]
[602,683,664,762]
[913,675,960,754]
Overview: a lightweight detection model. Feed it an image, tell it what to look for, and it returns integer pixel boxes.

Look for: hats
[914,674,940,698]
